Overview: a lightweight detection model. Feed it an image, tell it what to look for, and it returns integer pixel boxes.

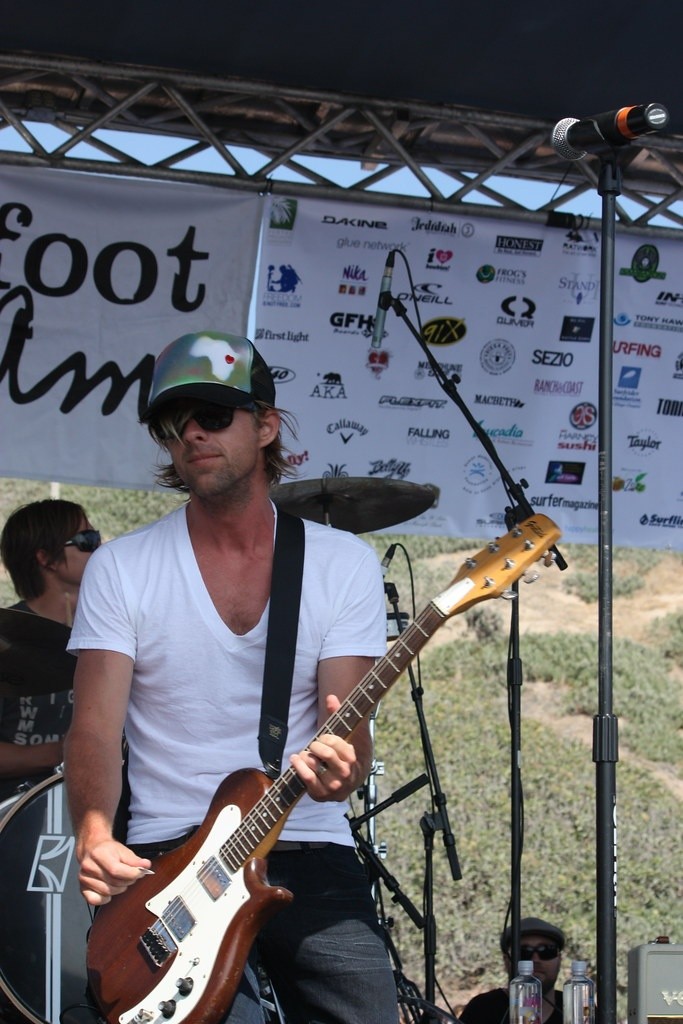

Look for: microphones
[550,103,669,160]
[380,542,398,578]
[371,249,395,348]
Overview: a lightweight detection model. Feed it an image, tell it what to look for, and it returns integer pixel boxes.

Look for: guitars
[84,513,561,1024]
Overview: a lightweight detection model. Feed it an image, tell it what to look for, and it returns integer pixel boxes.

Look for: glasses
[152,404,257,439]
[63,529,101,552]
[513,943,560,961]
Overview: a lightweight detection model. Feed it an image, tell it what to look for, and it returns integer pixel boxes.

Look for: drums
[0,772,102,1023]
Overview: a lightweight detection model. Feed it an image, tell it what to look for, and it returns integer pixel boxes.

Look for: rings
[317,765,328,774]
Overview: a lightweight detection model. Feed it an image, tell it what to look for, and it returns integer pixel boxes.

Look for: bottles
[508,960,544,1024]
[562,961,596,1024]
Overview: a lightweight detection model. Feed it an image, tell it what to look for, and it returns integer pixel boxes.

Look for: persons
[457,916,565,1024]
[0,499,100,799]
[453,1005,464,1018]
[62,332,398,1024]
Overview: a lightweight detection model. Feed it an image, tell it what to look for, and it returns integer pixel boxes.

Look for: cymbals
[268,476,436,535]
[0,607,79,698]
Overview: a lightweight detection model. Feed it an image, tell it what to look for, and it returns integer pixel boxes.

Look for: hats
[500,917,565,948]
[140,332,276,422]
